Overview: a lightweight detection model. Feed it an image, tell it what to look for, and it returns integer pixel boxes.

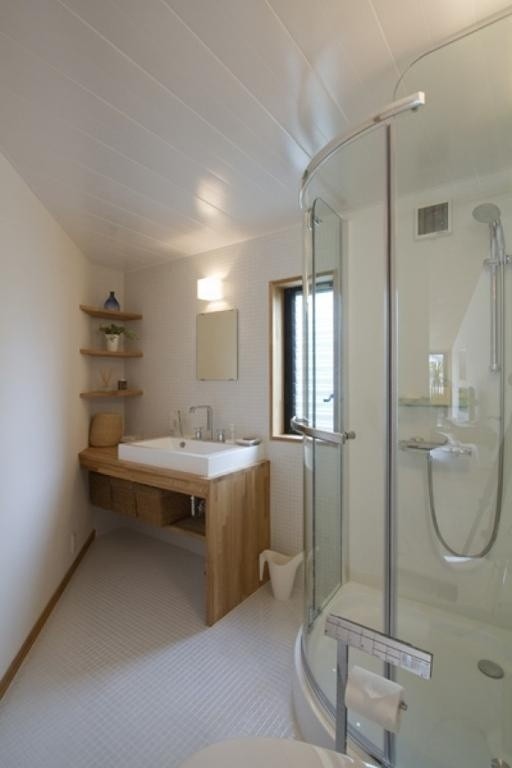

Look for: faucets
[188,405,214,441]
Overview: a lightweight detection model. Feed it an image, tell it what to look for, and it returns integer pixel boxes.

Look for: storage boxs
[134,481,163,526]
[88,469,112,510]
[111,477,137,518]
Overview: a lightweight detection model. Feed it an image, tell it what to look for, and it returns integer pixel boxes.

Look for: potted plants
[98,323,142,351]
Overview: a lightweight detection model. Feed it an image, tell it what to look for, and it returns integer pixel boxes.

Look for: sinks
[119,437,264,476]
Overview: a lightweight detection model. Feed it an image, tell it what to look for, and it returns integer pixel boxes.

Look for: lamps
[197,278,222,301]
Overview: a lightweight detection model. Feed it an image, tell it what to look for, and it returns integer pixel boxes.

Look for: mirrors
[195,308,238,381]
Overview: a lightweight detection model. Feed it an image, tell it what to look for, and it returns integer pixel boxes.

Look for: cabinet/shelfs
[78,445,270,627]
[80,305,143,399]
